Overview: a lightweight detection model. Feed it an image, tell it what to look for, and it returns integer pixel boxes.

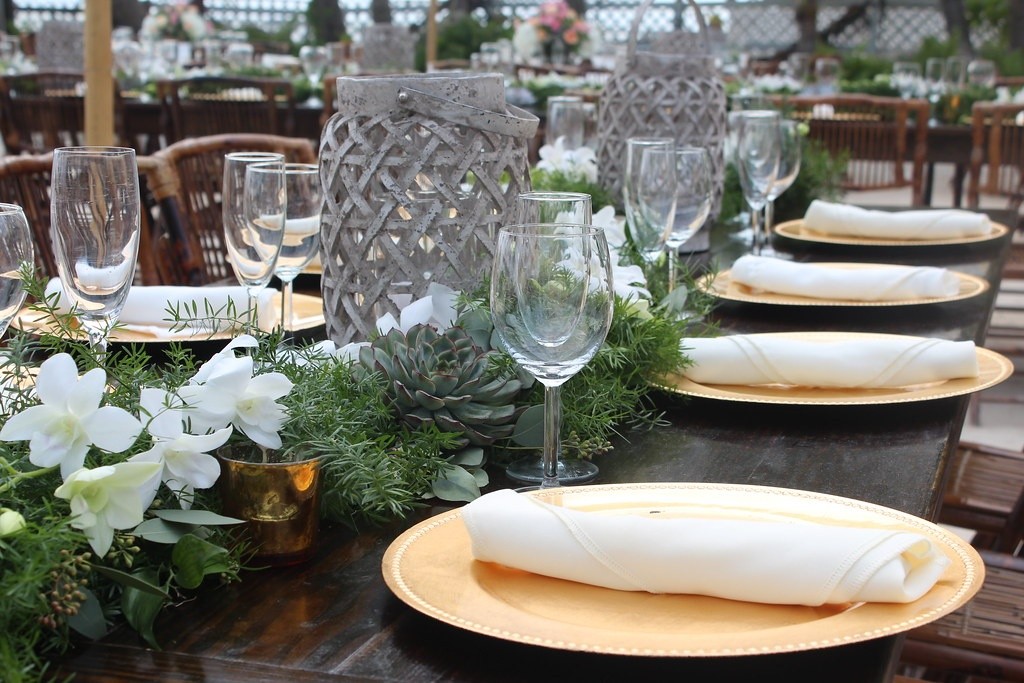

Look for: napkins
[455,486,982,609]
[670,331,980,391]
[40,266,284,333]
[734,256,968,299]
[801,200,994,243]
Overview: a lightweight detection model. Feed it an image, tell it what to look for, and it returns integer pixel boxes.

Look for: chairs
[0,76,323,304]
[898,443,1023,681]
[751,83,1024,192]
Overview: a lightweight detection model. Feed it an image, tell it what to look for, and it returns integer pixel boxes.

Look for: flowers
[0,141,732,681]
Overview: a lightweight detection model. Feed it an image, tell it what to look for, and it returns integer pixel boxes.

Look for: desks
[812,118,1024,201]
[115,91,323,139]
[40,199,1018,683]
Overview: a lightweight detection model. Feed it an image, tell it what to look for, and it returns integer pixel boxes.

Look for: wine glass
[222,151,288,358]
[488,108,811,493]
[122,29,342,88]
[240,163,324,350]
[783,52,997,121]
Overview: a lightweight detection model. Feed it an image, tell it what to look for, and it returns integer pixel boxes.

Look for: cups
[1,200,34,339]
[51,147,141,387]
[548,95,584,152]
[216,442,323,566]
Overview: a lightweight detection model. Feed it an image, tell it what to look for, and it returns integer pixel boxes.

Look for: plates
[773,211,1009,246]
[10,285,327,344]
[380,481,986,657]
[696,261,991,307]
[637,331,1015,404]
